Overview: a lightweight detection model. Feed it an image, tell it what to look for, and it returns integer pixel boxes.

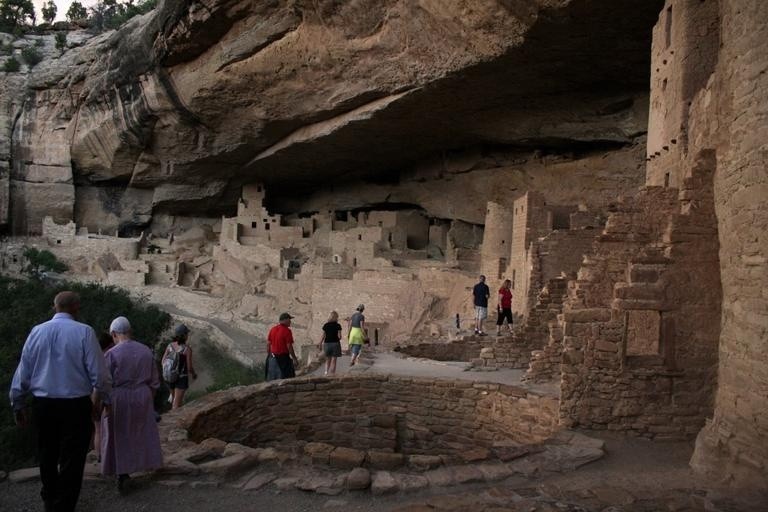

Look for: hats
[279,313,295,321]
[356,304,364,310]
[176,324,190,336]
[109,316,131,335]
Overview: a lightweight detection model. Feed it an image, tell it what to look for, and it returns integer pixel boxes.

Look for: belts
[271,355,289,358]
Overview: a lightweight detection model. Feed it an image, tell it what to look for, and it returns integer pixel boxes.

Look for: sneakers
[497,332,503,336]
[474,329,488,336]
[118,473,135,490]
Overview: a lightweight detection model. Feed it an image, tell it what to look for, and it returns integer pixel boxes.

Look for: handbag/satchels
[361,335,370,344]
[288,359,295,378]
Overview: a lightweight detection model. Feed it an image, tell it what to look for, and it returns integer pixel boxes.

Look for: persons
[265,313,299,379]
[161,324,198,409]
[9,291,161,512]
[472,275,489,336]
[347,304,367,366]
[496,279,513,336]
[320,310,342,376]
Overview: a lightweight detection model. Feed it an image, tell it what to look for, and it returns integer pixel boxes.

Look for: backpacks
[162,343,185,384]
[264,354,270,381]
